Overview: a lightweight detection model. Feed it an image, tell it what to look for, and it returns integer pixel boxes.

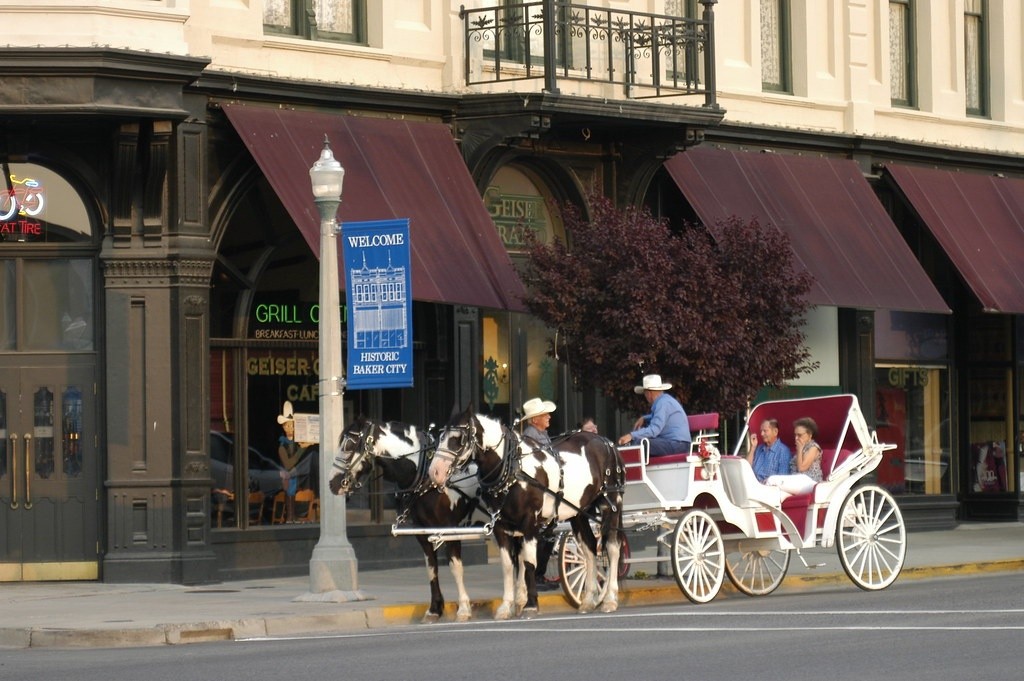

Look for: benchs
[644,413,718,470]
[719,450,853,508]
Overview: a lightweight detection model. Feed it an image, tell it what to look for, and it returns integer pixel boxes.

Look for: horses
[328,399,625,624]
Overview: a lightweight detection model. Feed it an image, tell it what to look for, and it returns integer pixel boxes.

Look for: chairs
[210,488,320,528]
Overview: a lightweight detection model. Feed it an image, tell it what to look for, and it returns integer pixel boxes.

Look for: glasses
[793,432,807,437]
[586,424,597,430]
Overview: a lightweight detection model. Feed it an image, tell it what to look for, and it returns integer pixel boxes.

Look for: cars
[210,429,288,520]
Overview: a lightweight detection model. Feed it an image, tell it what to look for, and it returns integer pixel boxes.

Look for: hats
[634,375,672,394]
[520,398,556,423]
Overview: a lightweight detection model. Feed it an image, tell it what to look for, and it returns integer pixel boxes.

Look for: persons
[514,396,562,592]
[764,416,824,535]
[570,415,609,590]
[619,372,693,457]
[744,416,792,485]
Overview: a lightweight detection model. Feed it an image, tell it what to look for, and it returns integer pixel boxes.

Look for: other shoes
[536,577,558,592]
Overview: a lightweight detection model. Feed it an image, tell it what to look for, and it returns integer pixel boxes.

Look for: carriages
[326,391,908,625]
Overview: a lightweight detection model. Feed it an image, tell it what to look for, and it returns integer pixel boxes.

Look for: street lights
[307,132,359,593]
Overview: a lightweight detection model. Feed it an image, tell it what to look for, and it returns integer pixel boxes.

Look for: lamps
[502,364,507,383]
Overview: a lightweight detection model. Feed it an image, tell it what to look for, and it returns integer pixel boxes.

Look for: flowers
[698,439,716,459]
